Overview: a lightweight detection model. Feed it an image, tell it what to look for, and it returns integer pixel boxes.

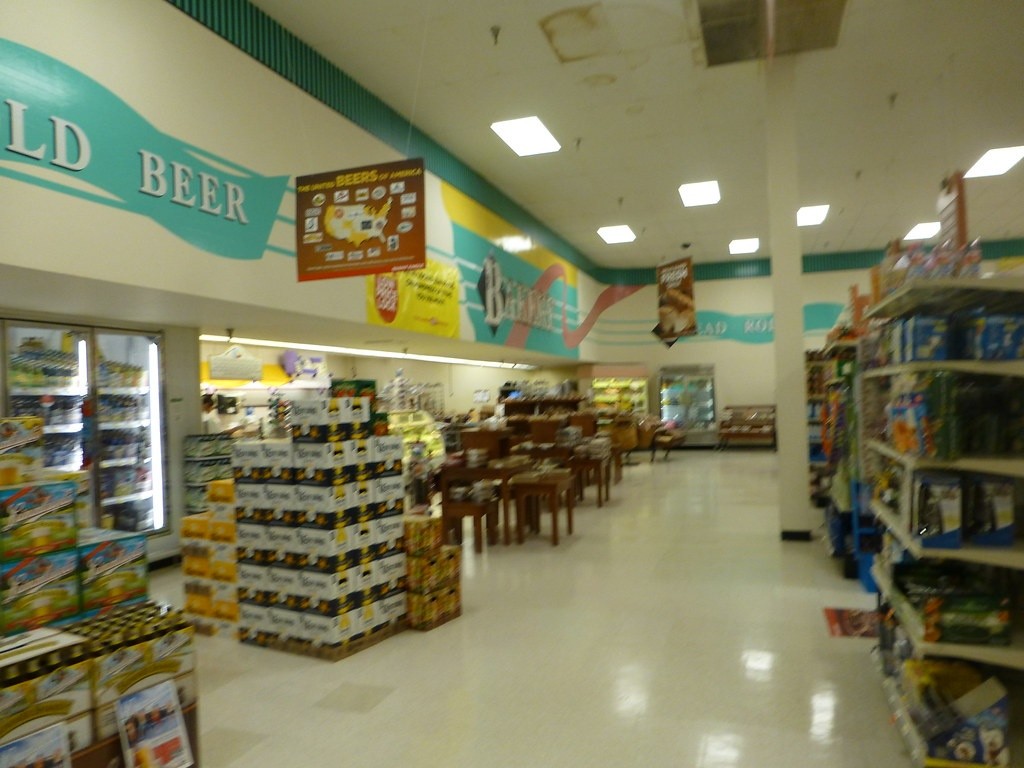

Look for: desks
[70,702,198,768]
[511,439,590,504]
[511,475,577,546]
[461,427,515,458]
[441,458,534,548]
[566,453,613,509]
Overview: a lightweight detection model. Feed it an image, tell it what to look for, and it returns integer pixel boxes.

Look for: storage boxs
[833,306,1024,766]
[0,415,45,486]
[44,468,93,531]
[89,619,196,709]
[182,378,462,660]
[0,480,78,563]
[66,711,92,754]
[76,527,149,612]
[0,548,81,636]
[0,627,93,745]
[91,670,198,743]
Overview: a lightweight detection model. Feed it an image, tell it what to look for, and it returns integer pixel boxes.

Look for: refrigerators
[656,365,718,446]
[0,308,174,562]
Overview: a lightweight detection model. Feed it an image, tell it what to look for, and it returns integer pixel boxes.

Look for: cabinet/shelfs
[857,277,1024,768]
[822,339,857,388]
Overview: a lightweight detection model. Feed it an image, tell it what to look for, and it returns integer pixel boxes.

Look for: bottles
[7,349,78,386]
[97,394,147,423]
[10,397,72,425]
[97,427,148,460]
[7,600,185,678]
[98,355,142,386]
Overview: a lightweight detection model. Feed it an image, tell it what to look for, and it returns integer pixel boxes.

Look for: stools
[440,497,501,554]
